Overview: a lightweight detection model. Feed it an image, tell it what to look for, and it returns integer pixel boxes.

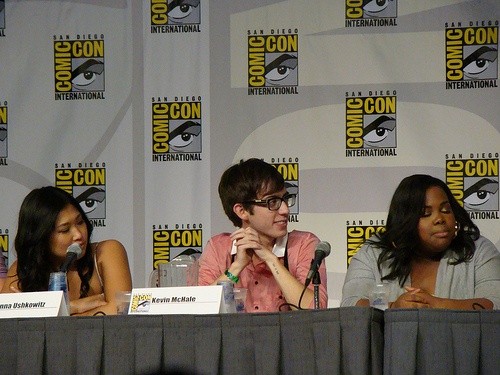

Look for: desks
[0,305,500,375]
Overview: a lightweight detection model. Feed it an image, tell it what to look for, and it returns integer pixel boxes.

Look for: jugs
[147,255,198,287]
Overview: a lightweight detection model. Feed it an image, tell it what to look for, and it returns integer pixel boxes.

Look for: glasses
[239,193,296,211]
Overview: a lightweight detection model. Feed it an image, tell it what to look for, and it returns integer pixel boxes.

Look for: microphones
[59,243,82,272]
[305,242,331,286]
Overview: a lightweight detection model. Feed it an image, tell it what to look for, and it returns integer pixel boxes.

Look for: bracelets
[224,268,239,283]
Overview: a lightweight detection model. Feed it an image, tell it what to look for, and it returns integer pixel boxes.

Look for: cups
[366,283,396,310]
[47,272,71,315]
[114,289,131,315]
[216,281,235,313]
[233,287,248,312]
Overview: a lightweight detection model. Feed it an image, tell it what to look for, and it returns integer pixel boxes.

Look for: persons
[186,158,328,313]
[0,186,133,317]
[340,175,500,311]
